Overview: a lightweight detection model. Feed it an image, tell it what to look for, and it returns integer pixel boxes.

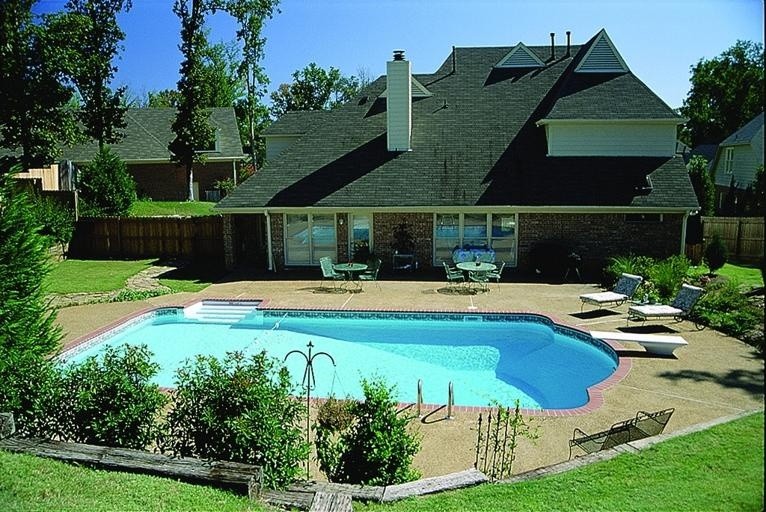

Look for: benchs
[567,407,675,462]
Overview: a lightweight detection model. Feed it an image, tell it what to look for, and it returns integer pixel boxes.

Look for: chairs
[443,259,506,296]
[355,258,383,292]
[579,273,710,333]
[319,256,347,292]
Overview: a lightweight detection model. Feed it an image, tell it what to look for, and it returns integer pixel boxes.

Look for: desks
[334,263,369,294]
